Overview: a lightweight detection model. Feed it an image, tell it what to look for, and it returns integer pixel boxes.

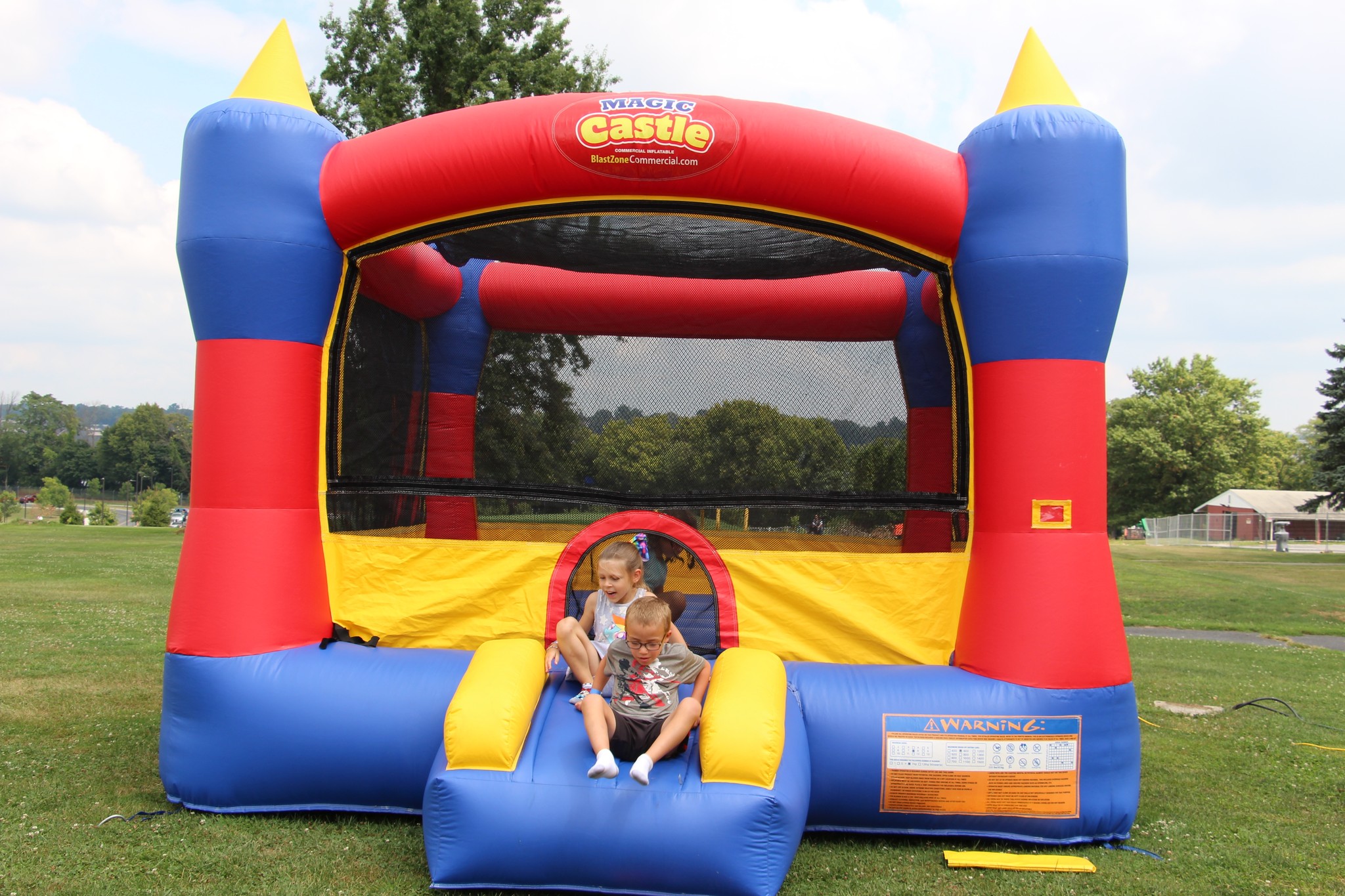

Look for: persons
[544,541,711,705]
[811,515,823,534]
[628,509,698,620]
[574,596,711,784]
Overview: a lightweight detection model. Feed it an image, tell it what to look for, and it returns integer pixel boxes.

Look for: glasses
[625,631,667,651]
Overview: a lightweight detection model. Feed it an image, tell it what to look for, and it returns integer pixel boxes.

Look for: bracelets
[590,688,601,695]
[548,641,559,646]
[546,646,561,654]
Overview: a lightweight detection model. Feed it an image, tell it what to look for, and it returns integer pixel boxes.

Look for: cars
[167,508,189,528]
[19,494,39,504]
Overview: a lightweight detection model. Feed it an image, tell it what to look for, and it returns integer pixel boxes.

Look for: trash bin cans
[1273,521,1291,551]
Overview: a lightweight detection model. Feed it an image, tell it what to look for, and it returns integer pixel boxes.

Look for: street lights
[101,478,105,526]
[167,467,173,493]
[151,471,158,490]
[135,472,145,505]
[126,479,136,526]
[139,475,150,514]
